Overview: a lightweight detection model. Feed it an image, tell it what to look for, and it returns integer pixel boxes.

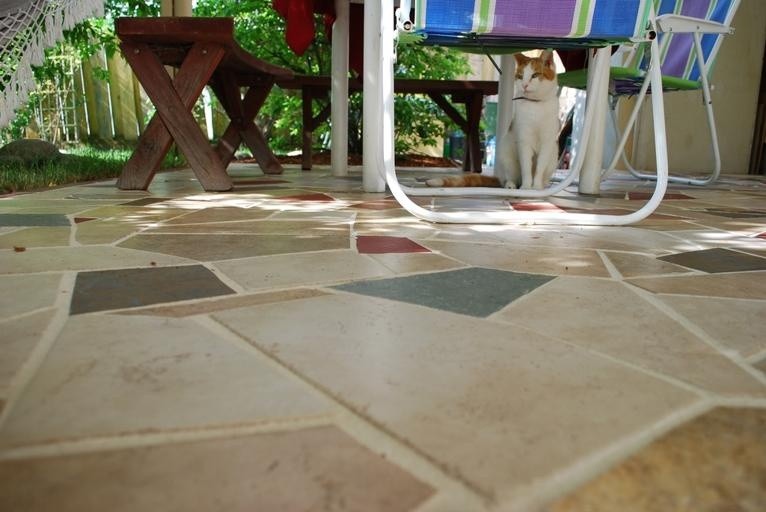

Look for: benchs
[114,15,292,193]
[274,76,498,174]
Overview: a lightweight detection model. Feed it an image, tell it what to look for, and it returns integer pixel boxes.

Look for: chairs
[555,1,742,186]
[379,0,669,226]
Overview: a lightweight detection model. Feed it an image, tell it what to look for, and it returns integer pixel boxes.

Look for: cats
[424,47,560,189]
